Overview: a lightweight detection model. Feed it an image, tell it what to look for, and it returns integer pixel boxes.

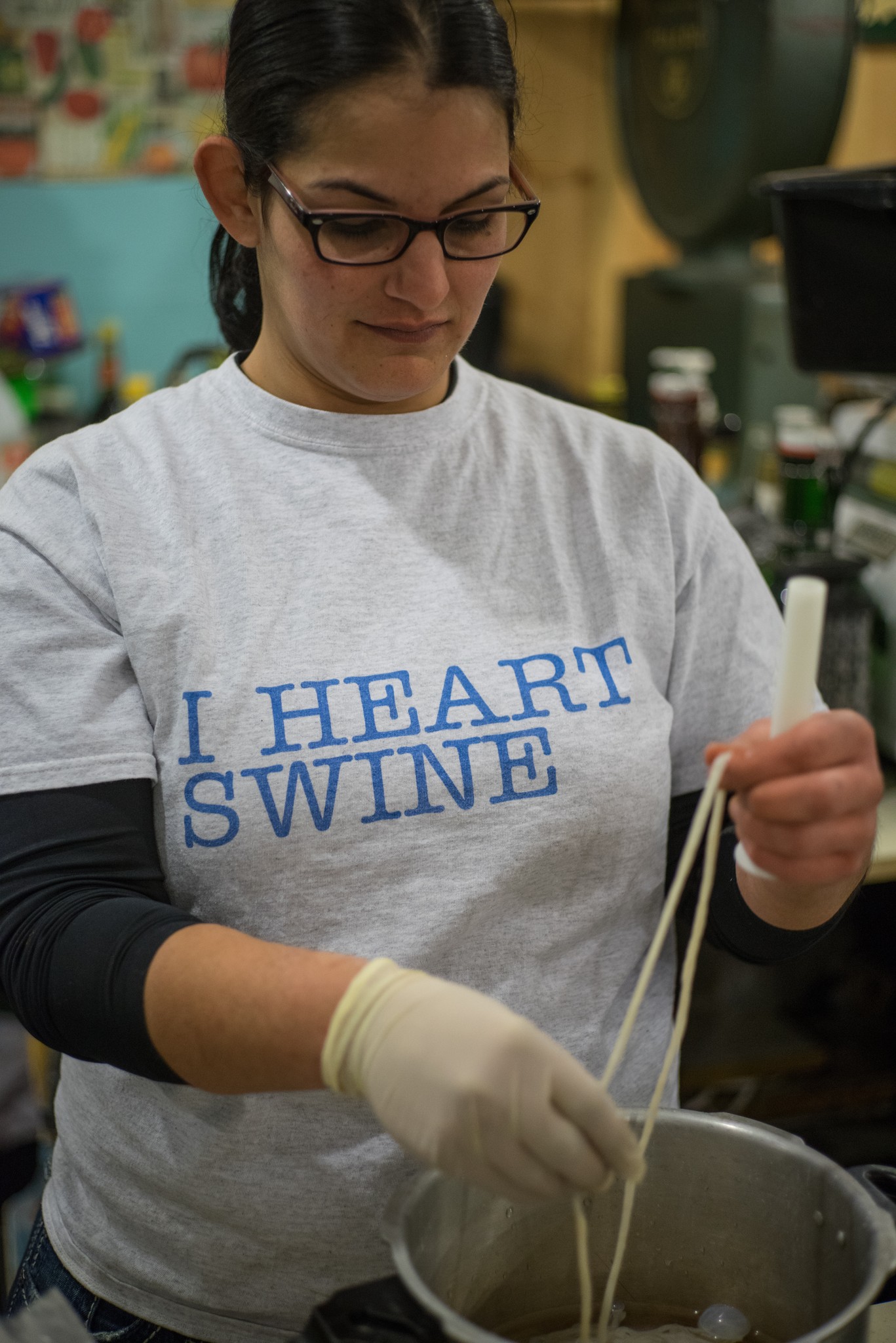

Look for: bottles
[644,345,895,780]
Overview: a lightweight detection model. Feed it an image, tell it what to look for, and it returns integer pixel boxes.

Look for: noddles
[536,749,732,1343]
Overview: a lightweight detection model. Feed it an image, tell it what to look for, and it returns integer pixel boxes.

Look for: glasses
[239,145,544,266]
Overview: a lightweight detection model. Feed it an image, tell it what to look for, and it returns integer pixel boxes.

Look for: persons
[0,0,880,1343]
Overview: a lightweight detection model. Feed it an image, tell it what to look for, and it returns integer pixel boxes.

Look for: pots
[370,1107,896,1343]
